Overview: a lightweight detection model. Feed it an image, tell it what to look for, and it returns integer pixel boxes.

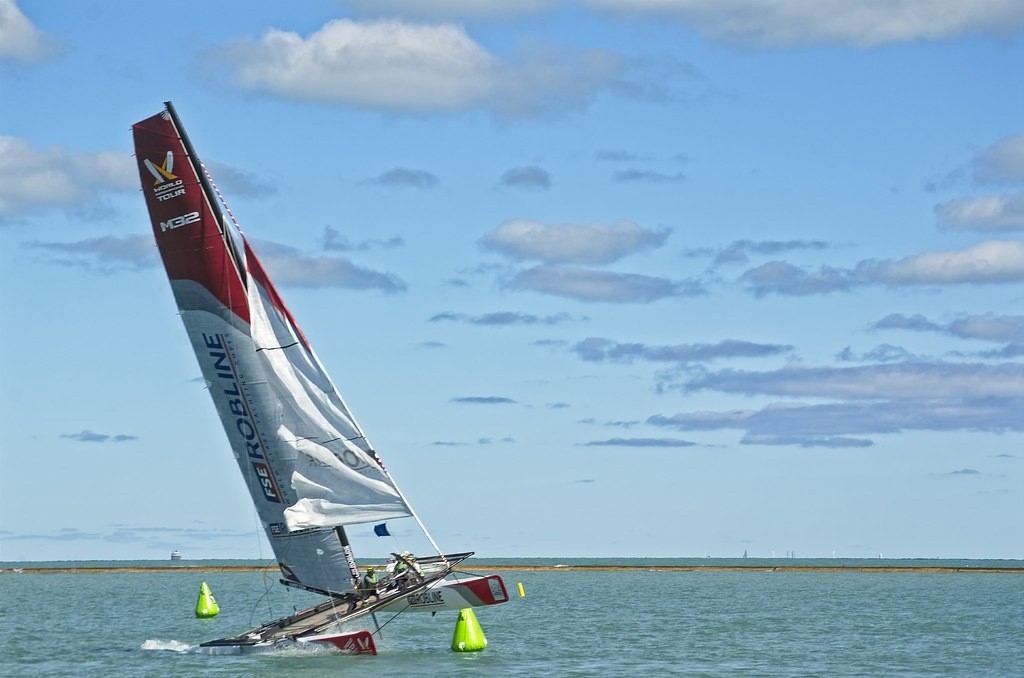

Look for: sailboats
[132,99,511,658]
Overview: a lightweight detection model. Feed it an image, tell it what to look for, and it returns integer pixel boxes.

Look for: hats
[404,552,409,556]
[407,554,415,560]
[367,567,375,573]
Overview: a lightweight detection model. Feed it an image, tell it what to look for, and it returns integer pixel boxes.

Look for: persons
[387,552,425,586]
[363,566,379,595]
